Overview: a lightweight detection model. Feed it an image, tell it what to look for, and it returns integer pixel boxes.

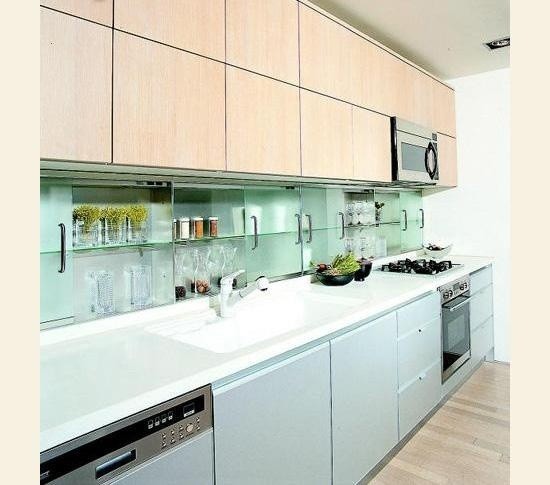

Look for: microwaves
[391,115,440,189]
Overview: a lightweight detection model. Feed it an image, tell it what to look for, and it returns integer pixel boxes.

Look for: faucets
[220,268,269,317]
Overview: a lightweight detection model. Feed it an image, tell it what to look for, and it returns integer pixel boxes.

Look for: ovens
[437,274,472,385]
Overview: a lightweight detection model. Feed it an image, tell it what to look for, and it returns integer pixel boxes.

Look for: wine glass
[345,202,369,261]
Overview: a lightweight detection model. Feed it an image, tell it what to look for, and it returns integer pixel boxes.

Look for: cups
[88,271,114,316]
[127,265,151,306]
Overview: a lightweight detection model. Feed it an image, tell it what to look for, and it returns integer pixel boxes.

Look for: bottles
[175,246,239,299]
[172,216,219,241]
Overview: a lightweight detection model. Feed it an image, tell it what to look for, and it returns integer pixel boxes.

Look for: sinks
[143,289,367,354]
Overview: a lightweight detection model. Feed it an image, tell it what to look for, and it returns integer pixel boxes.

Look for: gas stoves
[374,257,465,278]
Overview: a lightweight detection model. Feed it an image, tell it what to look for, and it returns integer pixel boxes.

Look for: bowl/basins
[352,262,372,282]
[316,274,354,286]
[424,245,451,259]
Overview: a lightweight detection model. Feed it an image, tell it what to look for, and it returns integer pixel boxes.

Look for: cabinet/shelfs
[470,254,497,380]
[112,1,226,189]
[438,132,458,191]
[225,1,300,189]
[173,188,247,307]
[300,89,393,186]
[244,185,302,290]
[344,188,401,264]
[399,191,425,254]
[43,185,175,335]
[396,292,443,451]
[40,0,112,183]
[301,186,346,278]
[211,313,399,485]
